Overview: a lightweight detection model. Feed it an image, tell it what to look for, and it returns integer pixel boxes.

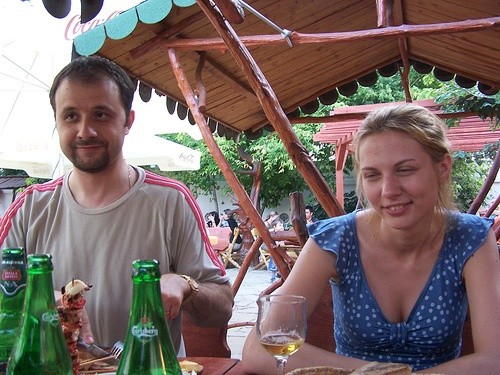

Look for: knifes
[77,336,120,366]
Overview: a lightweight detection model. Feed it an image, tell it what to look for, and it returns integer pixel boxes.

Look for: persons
[242,104,500,375]
[304,206,318,224]
[206,211,220,227]
[0,56,234,359]
[261,219,284,270]
[264,211,278,229]
[216,209,241,251]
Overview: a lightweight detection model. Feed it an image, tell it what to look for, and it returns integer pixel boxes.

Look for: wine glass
[256,295,307,375]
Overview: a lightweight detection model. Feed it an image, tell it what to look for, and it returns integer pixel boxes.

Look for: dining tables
[1,357,263,375]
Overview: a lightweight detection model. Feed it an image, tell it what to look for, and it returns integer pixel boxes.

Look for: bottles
[6,254,74,375]
[0,248,27,375]
[116,259,183,375]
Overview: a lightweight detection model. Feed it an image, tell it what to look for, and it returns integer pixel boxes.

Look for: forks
[110,340,124,359]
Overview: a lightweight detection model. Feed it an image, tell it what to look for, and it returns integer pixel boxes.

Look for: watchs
[180,275,199,306]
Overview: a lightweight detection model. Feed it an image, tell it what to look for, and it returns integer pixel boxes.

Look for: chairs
[216,227,243,271]
[250,228,277,271]
[274,244,305,261]
[170,286,235,360]
[228,237,243,264]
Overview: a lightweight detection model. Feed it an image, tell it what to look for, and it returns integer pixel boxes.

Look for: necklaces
[127,164,133,189]
